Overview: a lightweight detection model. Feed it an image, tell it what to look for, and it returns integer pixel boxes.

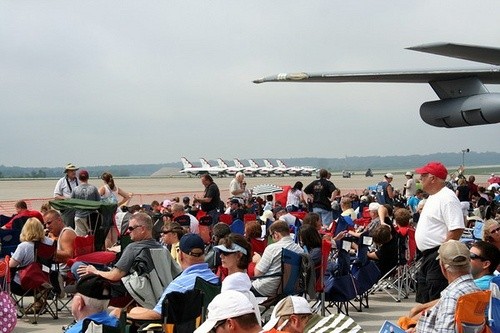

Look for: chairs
[0,207,492,333]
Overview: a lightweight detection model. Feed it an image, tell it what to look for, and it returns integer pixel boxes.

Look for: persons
[0,161,500,333]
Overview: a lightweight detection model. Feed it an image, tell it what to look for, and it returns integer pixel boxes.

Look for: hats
[183,196,190,201]
[64,274,112,300]
[192,290,255,333]
[415,161,448,181]
[213,242,247,255]
[262,295,312,331]
[175,232,205,257]
[63,162,79,173]
[435,239,470,266]
[405,171,413,177]
[360,196,369,202]
[365,202,381,212]
[79,169,89,179]
[162,199,174,208]
[487,183,500,191]
[385,172,393,179]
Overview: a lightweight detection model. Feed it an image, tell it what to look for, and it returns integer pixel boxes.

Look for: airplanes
[253,42,500,128]
[178,157,321,179]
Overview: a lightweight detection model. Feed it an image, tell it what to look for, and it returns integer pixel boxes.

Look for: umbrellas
[247,182,283,200]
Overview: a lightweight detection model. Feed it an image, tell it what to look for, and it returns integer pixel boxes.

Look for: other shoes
[23,306,46,315]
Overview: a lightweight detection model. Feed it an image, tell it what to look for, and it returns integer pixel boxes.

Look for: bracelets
[346,230,349,233]
[326,227,328,230]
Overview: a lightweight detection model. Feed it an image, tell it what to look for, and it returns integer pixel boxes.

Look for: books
[379,320,406,333]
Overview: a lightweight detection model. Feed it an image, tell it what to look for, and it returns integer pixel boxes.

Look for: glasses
[470,252,485,261]
[159,230,174,236]
[44,218,57,226]
[127,225,142,231]
[488,227,500,234]
[219,249,236,256]
[269,232,274,238]
